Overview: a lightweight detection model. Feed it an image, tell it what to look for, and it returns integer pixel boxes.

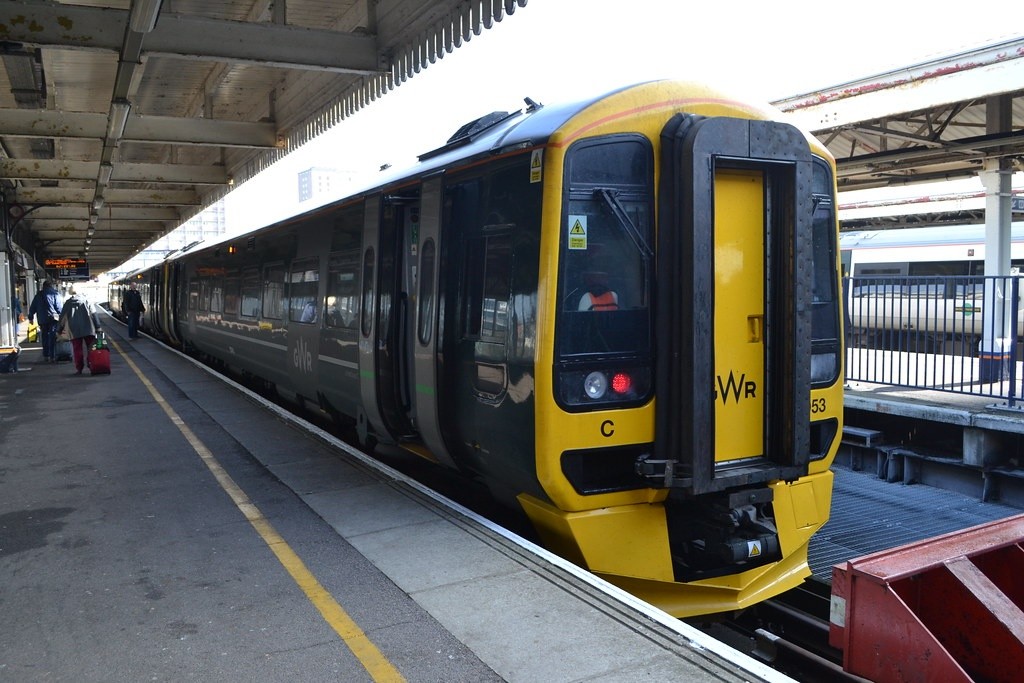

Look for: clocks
[8,204,24,219]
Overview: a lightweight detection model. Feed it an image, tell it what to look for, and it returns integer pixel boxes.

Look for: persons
[11,295,25,337]
[124,281,146,340]
[299,297,319,327]
[28,281,66,364]
[54,284,103,376]
[322,295,346,329]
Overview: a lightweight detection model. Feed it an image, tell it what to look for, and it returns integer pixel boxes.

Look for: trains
[106,73,850,618]
[839,220,1024,340]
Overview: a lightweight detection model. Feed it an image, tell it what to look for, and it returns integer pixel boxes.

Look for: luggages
[55,341,73,364]
[88,331,112,376]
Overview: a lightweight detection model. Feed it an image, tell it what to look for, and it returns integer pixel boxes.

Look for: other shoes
[73,371,84,377]
[44,357,51,363]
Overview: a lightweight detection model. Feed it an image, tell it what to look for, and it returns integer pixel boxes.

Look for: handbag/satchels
[51,314,60,321]
[18,313,25,322]
[56,325,70,341]
[27,321,38,343]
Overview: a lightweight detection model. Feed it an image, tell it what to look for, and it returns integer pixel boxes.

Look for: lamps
[93,196,104,209]
[90,214,98,224]
[129,0,162,32]
[98,165,113,184]
[84,228,95,256]
[107,101,131,138]
